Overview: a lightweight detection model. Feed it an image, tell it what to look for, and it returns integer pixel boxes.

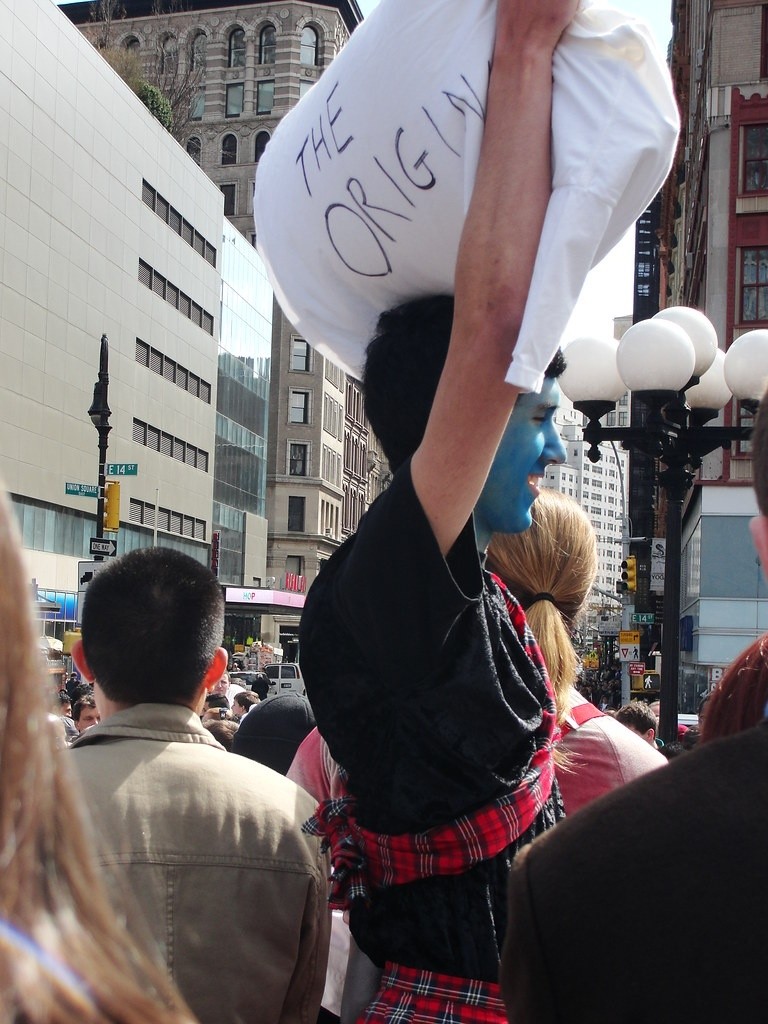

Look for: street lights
[87,333,114,561]
[556,306,768,745]
[587,441,648,707]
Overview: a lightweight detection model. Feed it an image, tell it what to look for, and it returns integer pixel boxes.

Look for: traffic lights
[620,555,637,592]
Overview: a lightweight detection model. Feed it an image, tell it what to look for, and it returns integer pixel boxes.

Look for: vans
[263,663,306,697]
[229,672,277,698]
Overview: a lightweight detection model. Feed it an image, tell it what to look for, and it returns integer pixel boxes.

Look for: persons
[501,385,767,1023]
[58,543,330,1024]
[573,657,714,762]
[45,664,315,778]
[298,0,579,1022]
[0,481,197,1024]
[282,487,668,1023]
[700,635,768,744]
